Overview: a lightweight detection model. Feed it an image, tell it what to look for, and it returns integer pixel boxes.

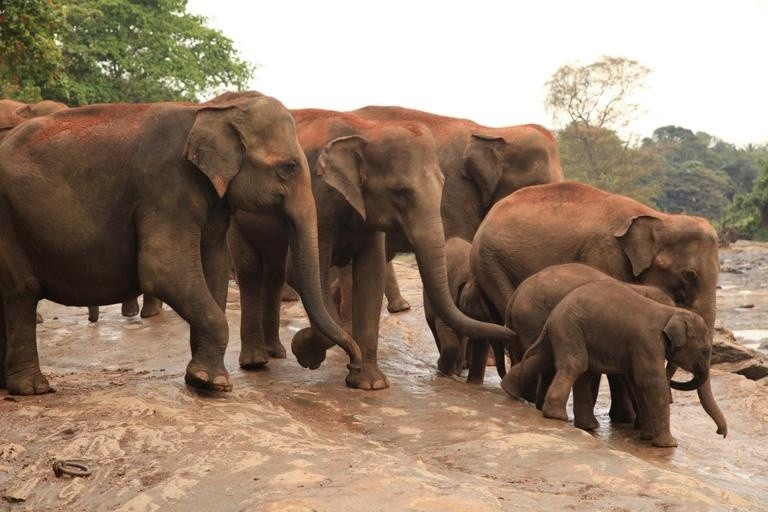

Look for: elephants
[0,88,728,449]
